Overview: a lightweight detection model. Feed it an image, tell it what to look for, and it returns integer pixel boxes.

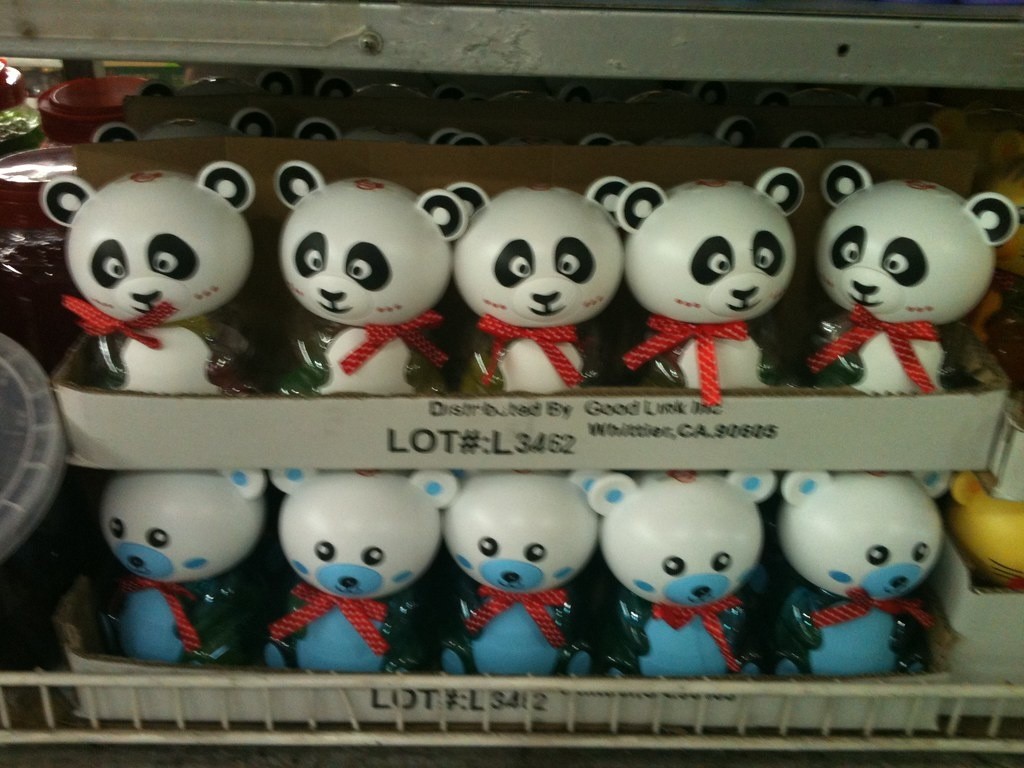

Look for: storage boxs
[48,142,1005,474]
[977,403,1024,502]
[931,540,1023,685]
[58,585,950,731]
